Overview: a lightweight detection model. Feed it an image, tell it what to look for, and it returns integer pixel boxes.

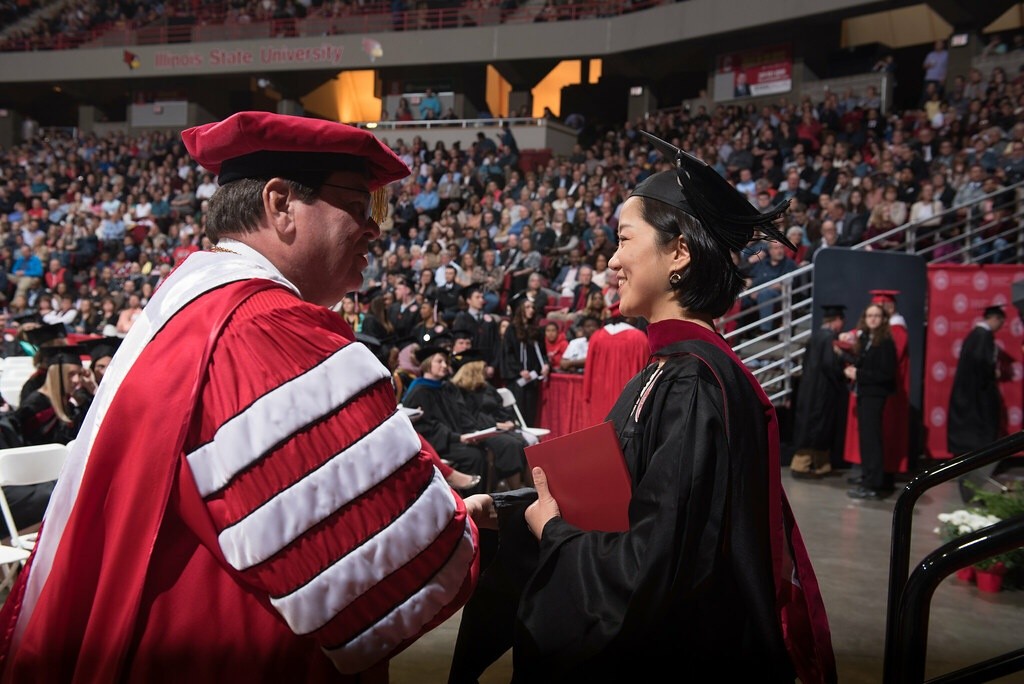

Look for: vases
[975,561,1007,593]
[955,565,973,584]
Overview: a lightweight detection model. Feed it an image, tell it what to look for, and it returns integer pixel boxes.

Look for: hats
[868,288,902,303]
[976,302,1009,317]
[13,310,123,366]
[820,302,848,316]
[628,129,799,254]
[180,111,413,195]
[342,266,654,366]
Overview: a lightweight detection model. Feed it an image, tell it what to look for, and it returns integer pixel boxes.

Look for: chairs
[0,442,67,551]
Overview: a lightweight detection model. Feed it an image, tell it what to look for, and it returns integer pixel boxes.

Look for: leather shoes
[847,474,886,501]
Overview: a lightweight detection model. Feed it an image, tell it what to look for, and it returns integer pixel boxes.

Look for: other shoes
[791,468,844,482]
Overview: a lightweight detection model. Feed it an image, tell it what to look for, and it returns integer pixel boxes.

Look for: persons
[394,342,422,403]
[544,321,569,370]
[561,316,600,371]
[377,343,482,490]
[833,305,895,498]
[834,293,909,484]
[455,287,499,388]
[239,1,1024,356]
[790,307,849,479]
[949,303,1007,478]
[99,127,479,684]
[461,167,797,683]
[402,349,491,497]
[499,297,545,424]
[0,0,237,540]
[454,354,537,491]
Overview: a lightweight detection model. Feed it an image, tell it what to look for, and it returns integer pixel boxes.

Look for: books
[523,420,635,535]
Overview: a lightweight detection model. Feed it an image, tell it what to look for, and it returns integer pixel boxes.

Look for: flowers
[934,509,1006,534]
